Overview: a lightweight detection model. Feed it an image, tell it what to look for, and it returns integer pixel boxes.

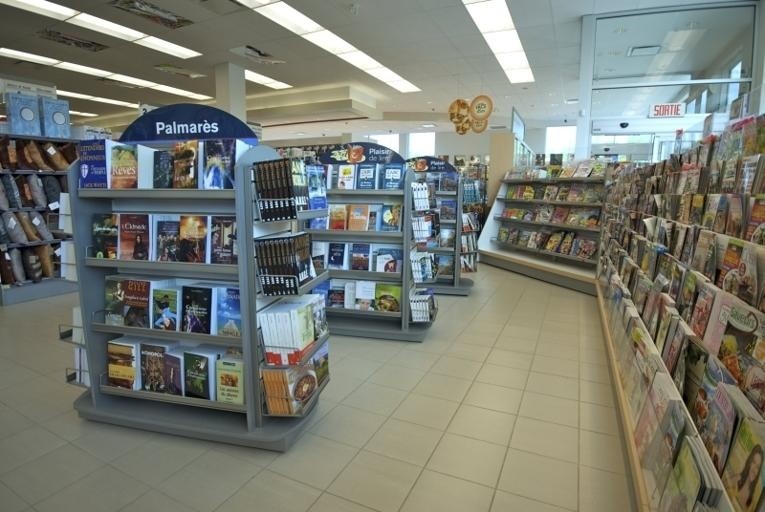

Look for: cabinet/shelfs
[486,132,537,220]
[50,136,332,453]
[0,130,91,308]
[399,155,485,297]
[275,142,437,343]
[596,111,765,512]
[477,162,608,297]
